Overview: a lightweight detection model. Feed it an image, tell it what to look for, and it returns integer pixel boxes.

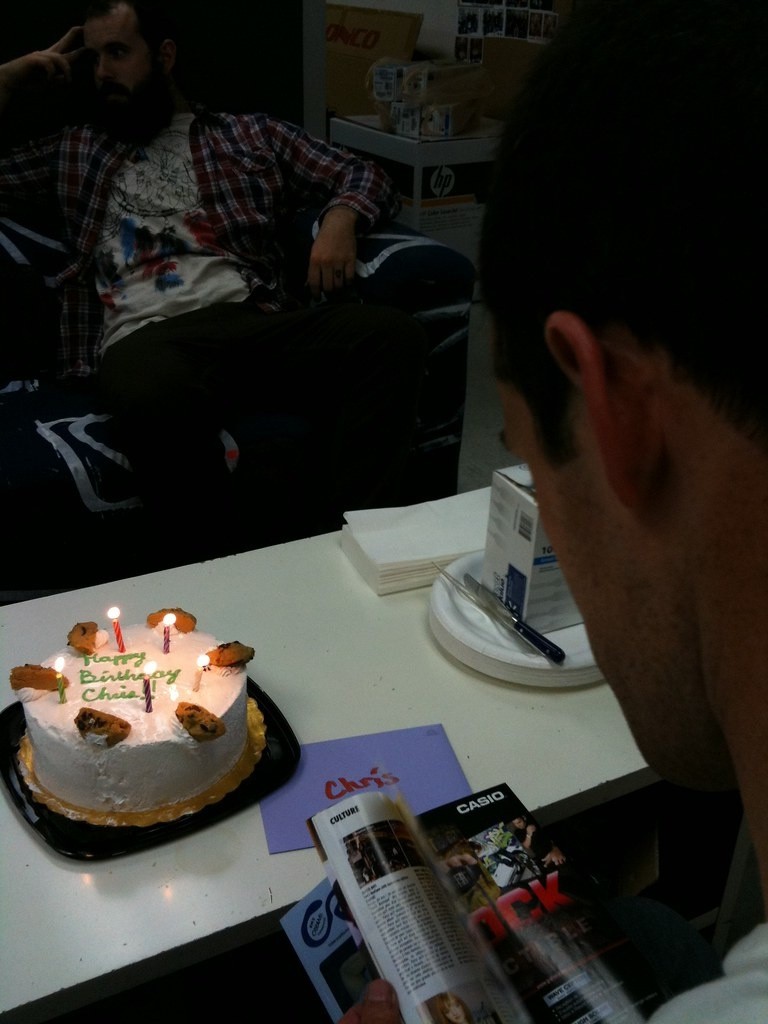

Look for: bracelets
[526,834,530,838]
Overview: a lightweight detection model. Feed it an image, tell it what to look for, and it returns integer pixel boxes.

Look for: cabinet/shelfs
[0,531,751,1023]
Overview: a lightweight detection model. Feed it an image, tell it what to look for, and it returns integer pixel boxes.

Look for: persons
[0,0,401,553]
[339,0,768,1024]
[511,816,566,868]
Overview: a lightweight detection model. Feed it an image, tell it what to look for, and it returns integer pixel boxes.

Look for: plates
[431,557,608,686]
[0,673,302,861]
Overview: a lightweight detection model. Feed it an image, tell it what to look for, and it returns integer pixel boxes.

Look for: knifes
[463,573,566,664]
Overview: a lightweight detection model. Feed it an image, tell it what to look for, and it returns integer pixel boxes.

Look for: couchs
[1,188,477,593]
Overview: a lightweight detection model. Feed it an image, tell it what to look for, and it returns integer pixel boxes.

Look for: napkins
[341,485,489,597]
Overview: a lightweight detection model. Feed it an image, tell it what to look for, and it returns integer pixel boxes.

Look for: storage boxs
[483,464,582,634]
[324,4,545,306]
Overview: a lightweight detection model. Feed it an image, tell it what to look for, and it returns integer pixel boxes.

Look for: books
[280,783,666,1024]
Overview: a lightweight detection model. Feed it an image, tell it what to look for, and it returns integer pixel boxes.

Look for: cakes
[9,607,256,813]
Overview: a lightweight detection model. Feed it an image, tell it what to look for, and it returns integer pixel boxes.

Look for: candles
[53,657,69,704]
[143,661,158,712]
[192,654,210,692]
[162,613,176,654]
[106,606,126,652]
